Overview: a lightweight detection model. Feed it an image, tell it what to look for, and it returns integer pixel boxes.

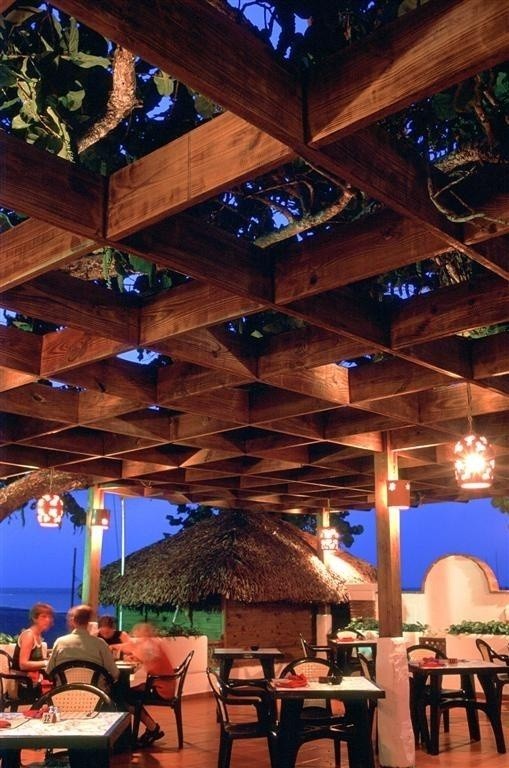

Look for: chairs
[28,684,113,768]
[406,645,450,734]
[46,661,115,713]
[299,632,334,673]
[0,648,45,713]
[206,668,280,767]
[39,657,132,706]
[129,650,194,752]
[476,638,509,718]
[279,658,346,768]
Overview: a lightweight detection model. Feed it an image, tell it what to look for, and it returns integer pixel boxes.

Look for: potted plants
[171,622,188,640]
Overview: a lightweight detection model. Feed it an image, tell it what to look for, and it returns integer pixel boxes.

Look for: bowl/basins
[329,676,343,684]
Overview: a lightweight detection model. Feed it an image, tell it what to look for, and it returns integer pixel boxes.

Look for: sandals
[137,722,165,747]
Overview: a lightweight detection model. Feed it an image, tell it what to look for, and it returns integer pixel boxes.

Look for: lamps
[455,432,496,489]
[38,494,64,530]
[89,509,110,532]
[385,479,410,510]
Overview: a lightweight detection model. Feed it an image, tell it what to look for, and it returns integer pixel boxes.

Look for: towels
[419,656,444,667]
[274,673,308,688]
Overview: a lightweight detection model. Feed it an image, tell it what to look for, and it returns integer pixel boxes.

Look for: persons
[46,604,121,705]
[123,622,176,748]
[6,603,55,703]
[92,615,132,661]
